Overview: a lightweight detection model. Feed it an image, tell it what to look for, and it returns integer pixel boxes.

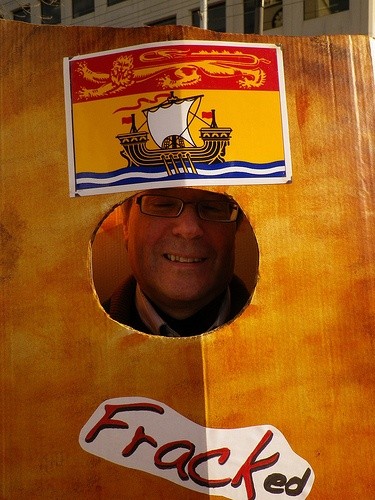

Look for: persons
[99,189,255,337]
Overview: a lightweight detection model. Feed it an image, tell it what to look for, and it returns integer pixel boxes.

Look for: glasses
[134,194,240,224]
